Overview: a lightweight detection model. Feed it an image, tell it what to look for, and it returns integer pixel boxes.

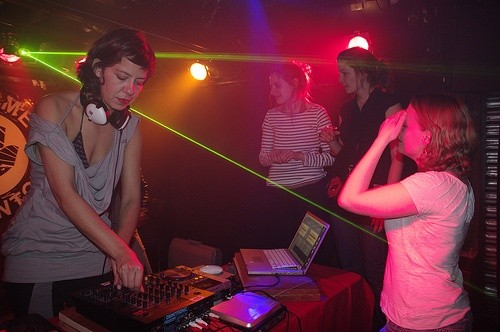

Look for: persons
[0,28,156,319]
[319,47,402,332]
[259,61,335,249]
[338,94,475,332]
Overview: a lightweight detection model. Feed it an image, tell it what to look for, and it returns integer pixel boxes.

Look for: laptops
[213,290,282,332]
[240,210,331,276]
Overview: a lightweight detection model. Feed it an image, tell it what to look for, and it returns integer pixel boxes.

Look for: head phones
[78,83,131,131]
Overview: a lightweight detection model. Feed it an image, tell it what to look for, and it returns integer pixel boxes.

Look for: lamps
[0,25,27,64]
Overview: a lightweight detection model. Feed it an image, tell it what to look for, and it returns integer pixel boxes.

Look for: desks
[47,261,376,332]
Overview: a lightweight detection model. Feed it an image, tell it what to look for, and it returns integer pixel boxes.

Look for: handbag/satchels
[168,237,222,269]
